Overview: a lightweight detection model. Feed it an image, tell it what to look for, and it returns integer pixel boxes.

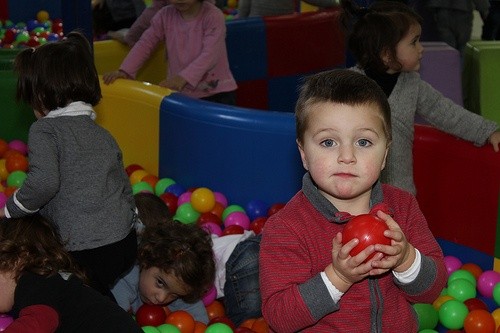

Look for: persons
[101,0,237,105]
[338,0,500,197]
[133,192,263,328]
[260,70,448,333]
[0,41,137,295]
[0,212,143,333]
[237,1,341,19]
[62,0,173,51]
[107,220,217,326]
[429,0,492,44]
